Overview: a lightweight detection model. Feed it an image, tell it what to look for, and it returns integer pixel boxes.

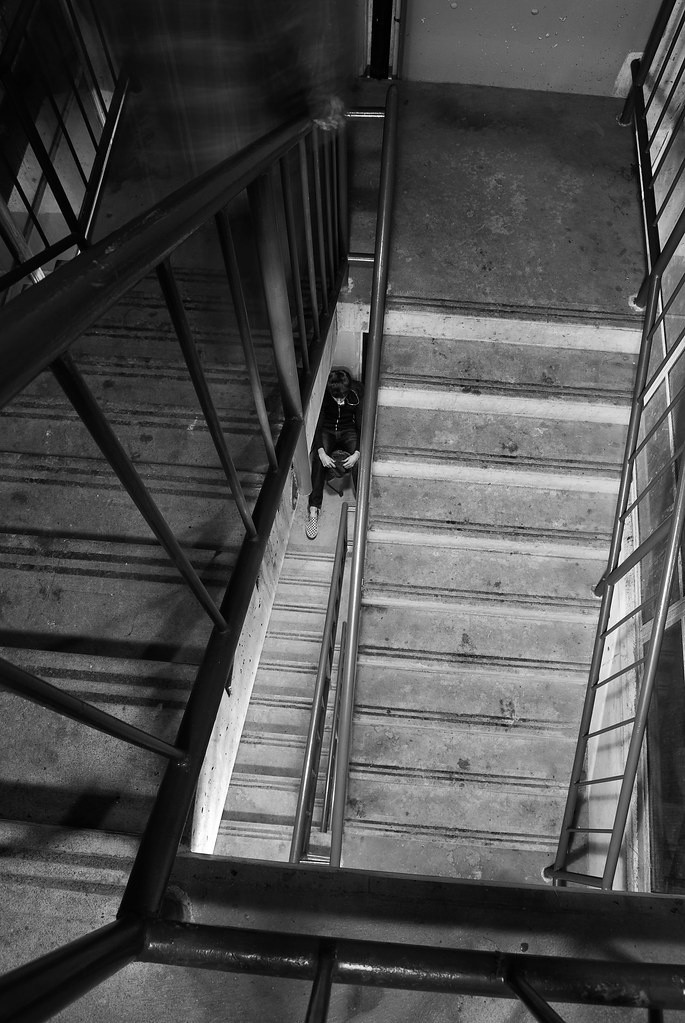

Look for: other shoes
[306,517,318,539]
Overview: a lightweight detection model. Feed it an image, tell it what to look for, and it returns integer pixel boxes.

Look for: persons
[305,369,361,540]
